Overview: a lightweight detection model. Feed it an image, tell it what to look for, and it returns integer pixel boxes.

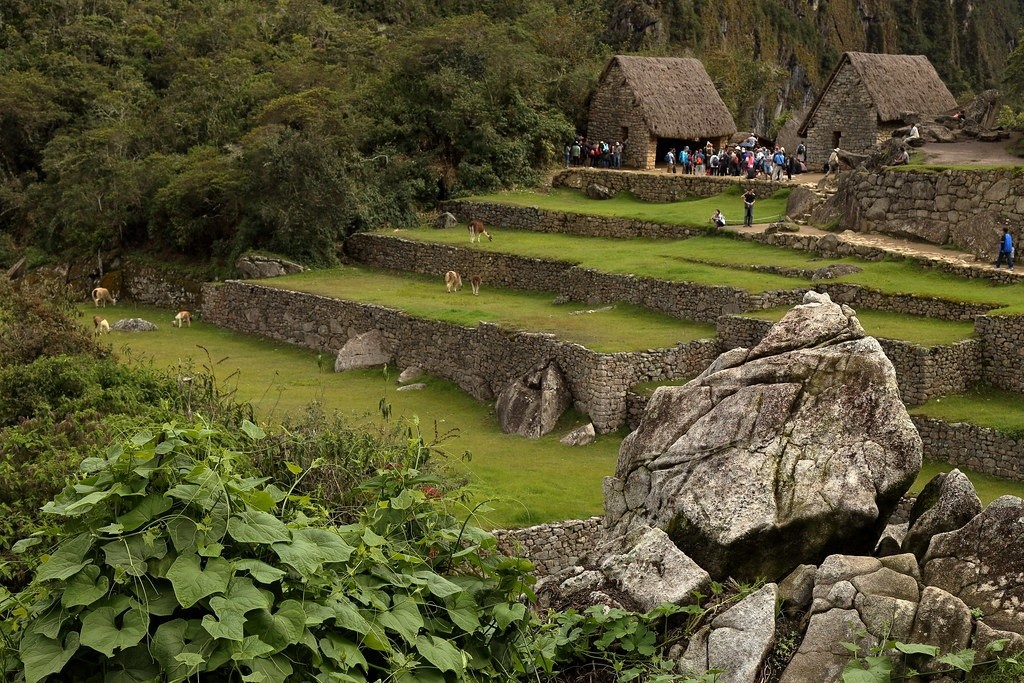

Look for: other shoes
[1006,267,1016,270]
[749,226,752,227]
[743,226,747,227]
[994,265,1000,268]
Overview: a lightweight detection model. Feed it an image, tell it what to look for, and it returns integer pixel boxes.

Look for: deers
[468,220,492,244]
[470,274,482,297]
[91,287,117,309]
[92,315,113,338]
[171,311,192,329]
[444,270,463,294]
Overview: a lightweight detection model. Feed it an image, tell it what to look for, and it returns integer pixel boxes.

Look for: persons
[740,188,756,227]
[903,123,920,143]
[953,110,966,120]
[887,147,910,166]
[664,133,807,182]
[994,228,1015,271]
[711,209,726,227]
[563,136,622,169]
[824,148,842,178]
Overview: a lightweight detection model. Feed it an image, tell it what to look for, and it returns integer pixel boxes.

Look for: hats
[780,147,784,152]
[616,142,619,145]
[735,146,741,150]
[576,141,578,143]
[834,148,840,152]
[742,147,745,152]
[751,133,754,135]
[590,142,595,145]
[762,146,766,149]
[600,141,604,143]
[775,146,779,150]
[757,148,762,150]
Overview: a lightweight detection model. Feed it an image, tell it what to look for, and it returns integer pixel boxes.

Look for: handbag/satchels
[782,163,786,170]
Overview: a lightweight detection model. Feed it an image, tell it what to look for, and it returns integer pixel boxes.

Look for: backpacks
[697,157,702,164]
[679,151,682,160]
[712,157,718,166]
[664,155,670,163]
[797,146,804,154]
[591,149,594,155]
